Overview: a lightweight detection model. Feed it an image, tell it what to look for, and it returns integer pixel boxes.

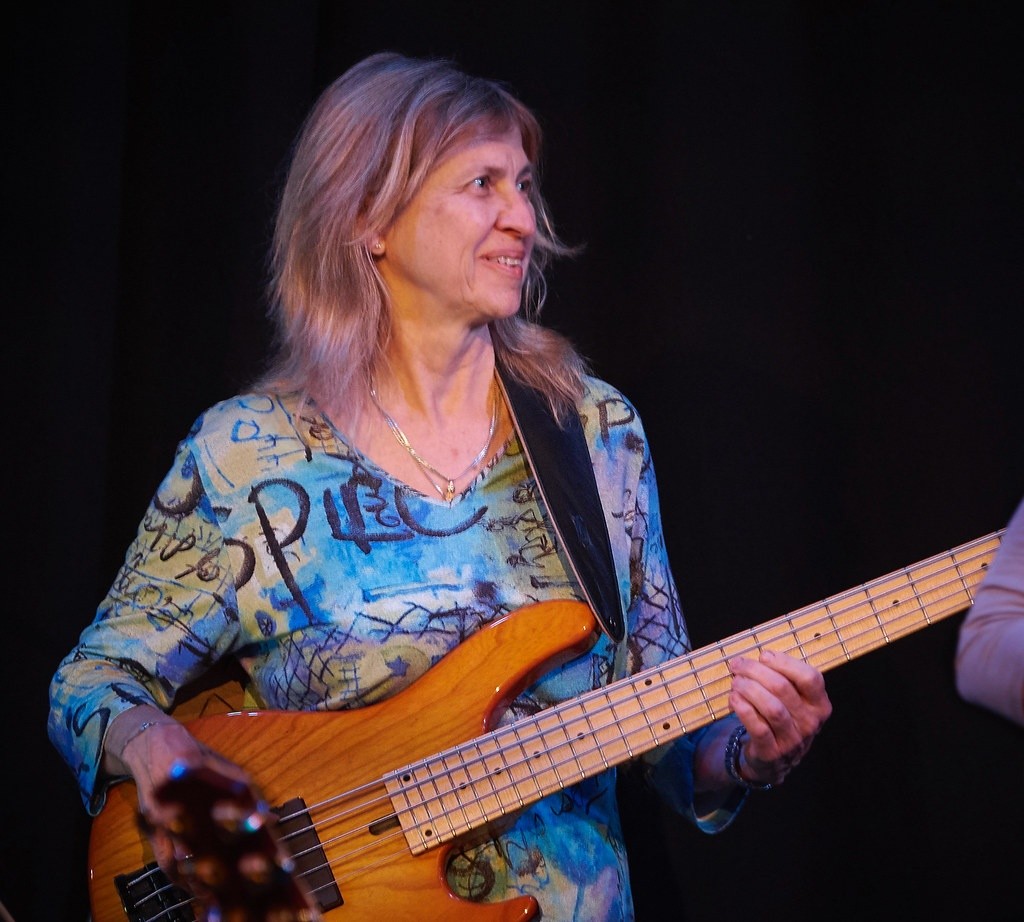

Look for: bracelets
[725,724,773,793]
[119,717,173,775]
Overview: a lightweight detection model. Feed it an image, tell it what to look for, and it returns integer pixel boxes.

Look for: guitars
[82,529,1011,922]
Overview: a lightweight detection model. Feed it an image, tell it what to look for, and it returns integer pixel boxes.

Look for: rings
[176,854,197,865]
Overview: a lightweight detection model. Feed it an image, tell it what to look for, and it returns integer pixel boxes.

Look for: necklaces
[364,374,501,501]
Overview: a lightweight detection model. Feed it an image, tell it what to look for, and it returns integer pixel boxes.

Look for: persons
[48,53,832,922]
[955,505,1024,722]
